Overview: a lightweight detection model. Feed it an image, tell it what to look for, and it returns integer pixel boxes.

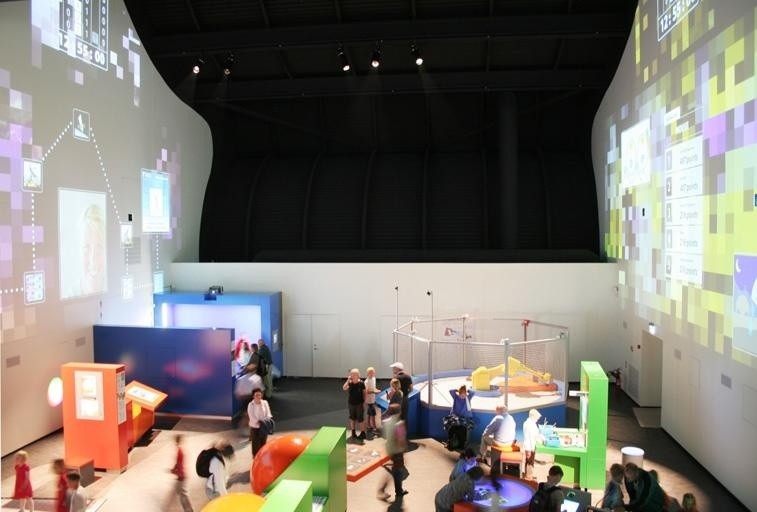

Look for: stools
[500,450,523,478]
[64,456,95,488]
[621,446,646,468]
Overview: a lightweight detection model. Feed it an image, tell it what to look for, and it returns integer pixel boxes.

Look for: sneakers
[395,489,408,496]
[377,493,391,501]
[350,429,357,439]
[366,427,381,435]
[357,430,366,439]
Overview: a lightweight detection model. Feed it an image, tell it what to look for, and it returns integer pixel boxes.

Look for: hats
[388,361,403,370]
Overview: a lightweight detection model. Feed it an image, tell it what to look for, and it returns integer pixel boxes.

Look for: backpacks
[195,446,226,479]
[254,352,267,377]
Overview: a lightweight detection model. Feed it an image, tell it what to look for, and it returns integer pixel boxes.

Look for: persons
[65,473,88,512]
[247,388,273,459]
[204,438,234,501]
[476,406,516,463]
[538,465,564,512]
[435,448,484,512]
[343,367,381,439]
[161,434,195,512]
[524,409,541,479]
[377,363,413,498]
[12,451,34,512]
[234,338,273,400]
[601,462,697,512]
[53,459,71,512]
[450,386,476,420]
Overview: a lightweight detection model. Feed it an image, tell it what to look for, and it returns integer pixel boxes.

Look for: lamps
[203,286,223,301]
